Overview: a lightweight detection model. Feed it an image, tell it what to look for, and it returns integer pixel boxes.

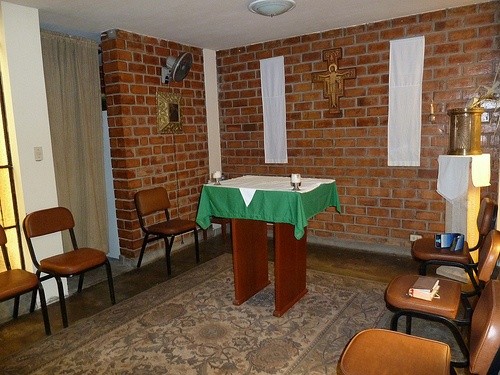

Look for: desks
[196,175,341,317]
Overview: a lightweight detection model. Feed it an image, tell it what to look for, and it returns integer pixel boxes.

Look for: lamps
[248,0,296,17]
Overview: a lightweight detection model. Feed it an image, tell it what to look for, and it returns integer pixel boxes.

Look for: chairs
[0,225,51,335]
[134,187,199,274]
[23,206,115,328]
[411,198,498,297]
[337,279,500,375]
[384,229,500,360]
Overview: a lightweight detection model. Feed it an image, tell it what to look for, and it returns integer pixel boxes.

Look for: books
[405,275,441,301]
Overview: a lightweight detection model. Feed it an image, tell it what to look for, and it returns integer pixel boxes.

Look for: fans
[161,52,192,83]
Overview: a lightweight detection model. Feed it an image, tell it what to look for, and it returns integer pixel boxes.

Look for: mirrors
[155,92,186,134]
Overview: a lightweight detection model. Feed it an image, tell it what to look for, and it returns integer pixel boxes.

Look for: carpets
[0,252,467,375]
